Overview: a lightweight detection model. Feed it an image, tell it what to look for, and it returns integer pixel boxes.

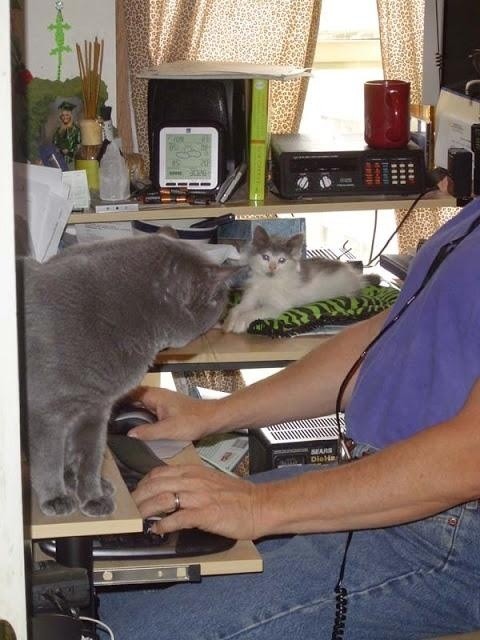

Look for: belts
[336,434,369,464]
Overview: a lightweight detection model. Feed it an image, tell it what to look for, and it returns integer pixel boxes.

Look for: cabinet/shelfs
[64,182,458,372]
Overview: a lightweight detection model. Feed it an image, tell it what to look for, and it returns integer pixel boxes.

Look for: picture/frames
[14,68,109,173]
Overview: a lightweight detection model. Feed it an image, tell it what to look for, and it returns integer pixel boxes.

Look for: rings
[173,491,181,511]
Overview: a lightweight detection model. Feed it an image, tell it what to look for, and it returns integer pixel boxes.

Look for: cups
[362,80,411,151]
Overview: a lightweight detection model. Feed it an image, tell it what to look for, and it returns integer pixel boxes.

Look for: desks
[28,436,265,638]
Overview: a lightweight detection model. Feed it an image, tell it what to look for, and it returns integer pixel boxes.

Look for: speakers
[147,79,246,191]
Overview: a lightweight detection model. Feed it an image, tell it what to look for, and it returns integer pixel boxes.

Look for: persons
[53,101,81,163]
[98,194,480,639]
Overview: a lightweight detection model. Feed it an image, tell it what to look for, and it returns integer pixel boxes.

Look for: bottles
[75,118,107,199]
[39,142,69,173]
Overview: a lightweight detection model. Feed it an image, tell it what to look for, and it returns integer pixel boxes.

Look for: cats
[218,224,384,335]
[24,224,250,520]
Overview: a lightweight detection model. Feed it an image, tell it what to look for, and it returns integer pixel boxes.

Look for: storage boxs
[433,79,480,182]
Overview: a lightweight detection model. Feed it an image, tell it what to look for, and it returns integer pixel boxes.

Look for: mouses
[109,406,157,434]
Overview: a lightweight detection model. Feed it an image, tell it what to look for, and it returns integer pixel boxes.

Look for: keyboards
[38,434,238,563]
